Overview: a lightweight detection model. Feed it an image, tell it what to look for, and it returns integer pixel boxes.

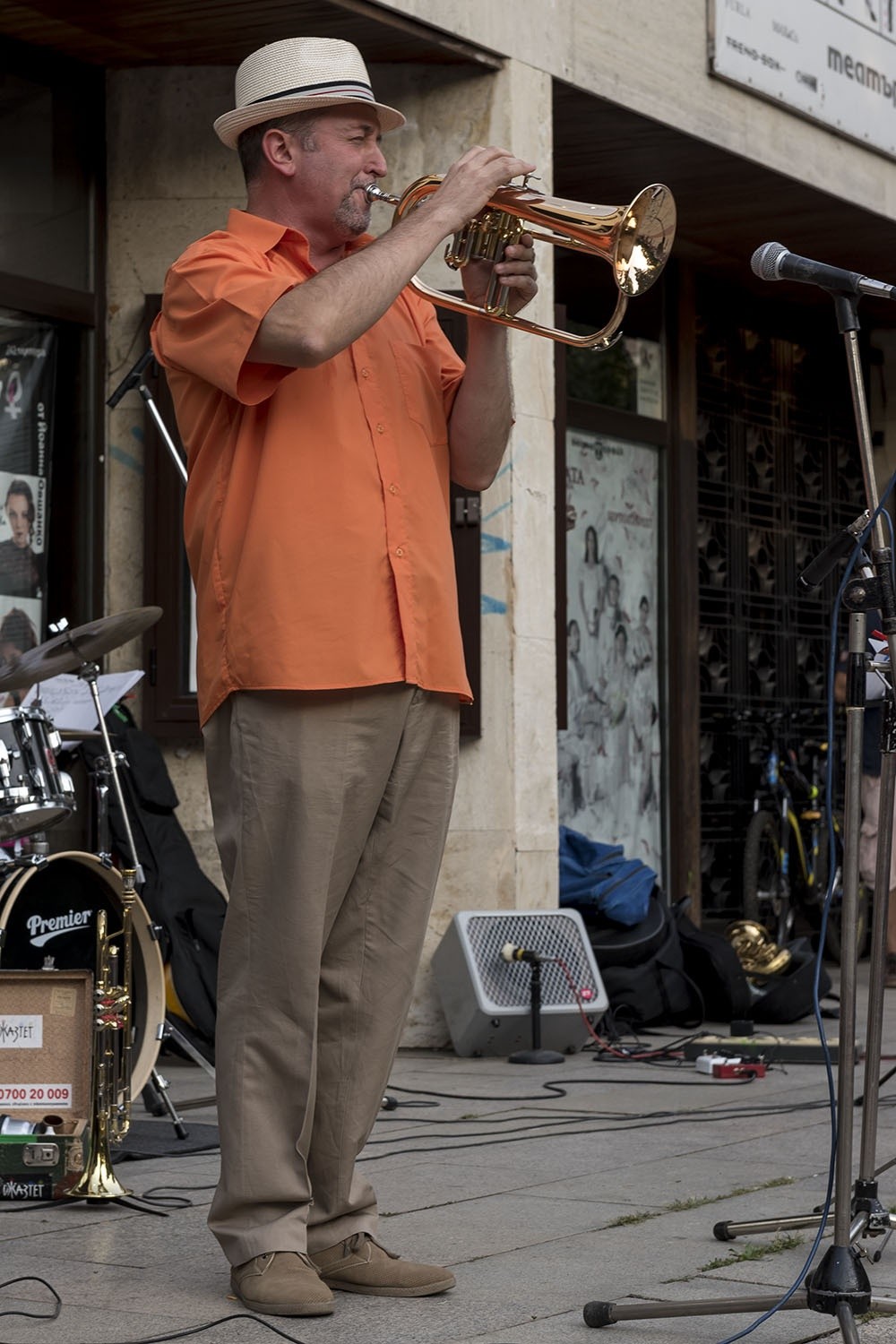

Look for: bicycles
[716,704,871,967]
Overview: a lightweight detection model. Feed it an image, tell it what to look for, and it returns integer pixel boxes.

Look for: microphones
[750,242,896,299]
[501,944,555,962]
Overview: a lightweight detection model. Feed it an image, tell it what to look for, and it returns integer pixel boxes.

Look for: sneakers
[230,1252,335,1316]
[309,1233,457,1297]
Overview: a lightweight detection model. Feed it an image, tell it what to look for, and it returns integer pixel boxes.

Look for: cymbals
[0,607,163,694]
[52,725,118,741]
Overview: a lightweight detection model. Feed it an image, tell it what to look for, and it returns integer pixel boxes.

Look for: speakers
[431,909,610,1058]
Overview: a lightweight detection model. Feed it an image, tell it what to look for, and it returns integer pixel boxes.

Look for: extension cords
[695,1051,767,1074]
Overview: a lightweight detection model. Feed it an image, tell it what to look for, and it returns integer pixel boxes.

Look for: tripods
[583,304,896,1343]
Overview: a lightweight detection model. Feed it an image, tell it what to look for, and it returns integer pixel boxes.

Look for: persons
[146,33,541,1316]
[0,607,38,708]
[558,526,663,890]
[0,478,45,598]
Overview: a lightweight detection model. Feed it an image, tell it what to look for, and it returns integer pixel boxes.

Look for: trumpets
[61,870,136,1198]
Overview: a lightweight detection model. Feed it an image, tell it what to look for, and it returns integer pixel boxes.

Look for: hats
[212,37,405,149]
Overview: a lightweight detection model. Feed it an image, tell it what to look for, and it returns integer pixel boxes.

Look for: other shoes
[882,952,896,988]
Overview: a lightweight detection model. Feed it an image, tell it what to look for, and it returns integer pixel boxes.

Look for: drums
[0,851,165,1121]
[0,706,73,844]
